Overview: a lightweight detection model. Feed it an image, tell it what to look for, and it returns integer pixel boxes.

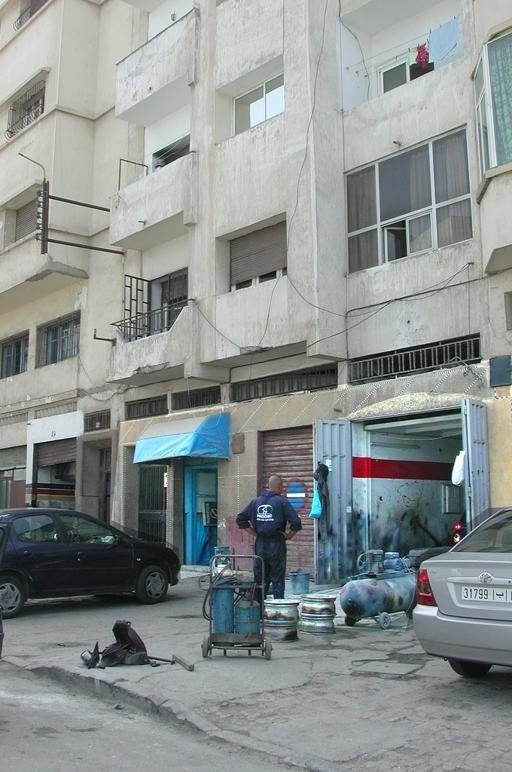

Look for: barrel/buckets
[290,569,311,595]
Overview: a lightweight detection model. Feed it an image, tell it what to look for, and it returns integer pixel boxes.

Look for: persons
[237,476,303,600]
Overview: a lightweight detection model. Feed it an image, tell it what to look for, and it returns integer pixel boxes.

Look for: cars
[414,505,512,678]
[0,508,181,619]
